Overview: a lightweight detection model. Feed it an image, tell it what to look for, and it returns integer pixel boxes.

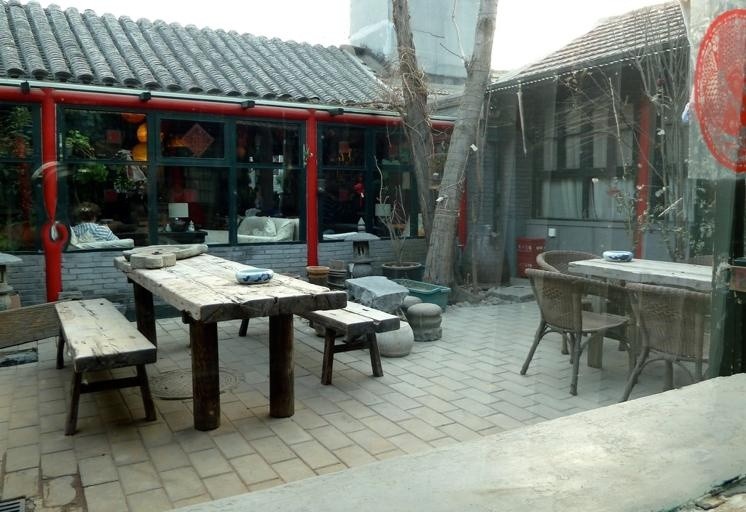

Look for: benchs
[239,300,399,387]
[54,298,158,438]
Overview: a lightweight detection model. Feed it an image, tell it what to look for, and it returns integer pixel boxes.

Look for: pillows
[261,217,277,236]
[271,221,295,242]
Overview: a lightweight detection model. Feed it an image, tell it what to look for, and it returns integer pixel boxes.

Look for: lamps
[168,202,189,231]
[375,203,392,228]
[132,117,163,161]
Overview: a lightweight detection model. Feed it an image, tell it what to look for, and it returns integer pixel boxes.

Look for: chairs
[519,267,638,396]
[617,282,713,405]
[536,249,628,354]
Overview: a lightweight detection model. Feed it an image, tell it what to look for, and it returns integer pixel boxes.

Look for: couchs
[201,216,300,244]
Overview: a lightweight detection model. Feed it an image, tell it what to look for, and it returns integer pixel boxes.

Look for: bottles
[188,220,195,232]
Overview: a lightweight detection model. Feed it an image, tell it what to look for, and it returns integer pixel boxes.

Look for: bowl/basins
[237,269,273,285]
[602,250,633,262]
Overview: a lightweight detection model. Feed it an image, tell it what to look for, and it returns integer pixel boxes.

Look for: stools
[311,321,346,338]
[374,320,414,358]
[399,295,423,318]
[408,302,443,342]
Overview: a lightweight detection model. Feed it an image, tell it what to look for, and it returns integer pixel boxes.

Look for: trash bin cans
[517,237,545,279]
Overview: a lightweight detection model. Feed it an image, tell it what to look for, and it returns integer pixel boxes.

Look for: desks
[342,276,409,342]
[112,226,208,250]
[373,226,391,237]
[114,252,348,432]
[569,258,713,369]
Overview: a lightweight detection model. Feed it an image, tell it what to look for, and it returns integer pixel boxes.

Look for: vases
[392,278,452,310]
[382,261,421,282]
